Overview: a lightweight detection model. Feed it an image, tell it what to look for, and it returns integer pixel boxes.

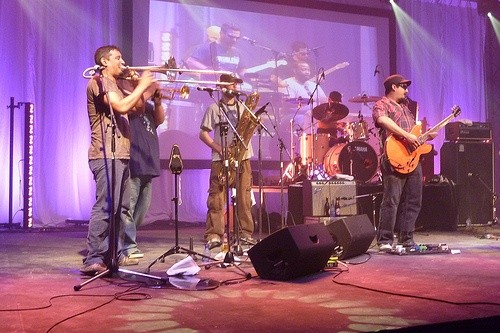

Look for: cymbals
[285,97,315,105]
[348,96,382,104]
[312,102,349,123]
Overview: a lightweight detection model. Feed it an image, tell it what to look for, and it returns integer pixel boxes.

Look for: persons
[180,25,329,135]
[372,74,439,253]
[199,73,264,250]
[79,45,165,275]
[315,90,342,142]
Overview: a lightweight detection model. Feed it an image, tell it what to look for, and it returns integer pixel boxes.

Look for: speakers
[356,184,383,227]
[327,214,376,260]
[439,140,495,226]
[412,182,457,231]
[247,223,334,282]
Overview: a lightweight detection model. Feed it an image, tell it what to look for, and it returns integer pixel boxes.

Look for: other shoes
[118,257,139,265]
[241,236,258,244]
[127,246,144,258]
[85,263,102,270]
[380,243,392,251]
[398,240,415,246]
[208,237,221,250]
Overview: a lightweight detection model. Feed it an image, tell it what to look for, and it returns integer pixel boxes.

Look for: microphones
[322,69,325,80]
[373,66,377,76]
[94,66,105,71]
[222,88,241,95]
[197,86,218,91]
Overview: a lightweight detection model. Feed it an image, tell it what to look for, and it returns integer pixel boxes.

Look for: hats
[220,74,243,84]
[384,74,411,86]
[330,91,341,102]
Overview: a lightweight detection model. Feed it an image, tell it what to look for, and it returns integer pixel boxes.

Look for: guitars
[310,62,351,81]
[240,60,288,79]
[383,105,462,174]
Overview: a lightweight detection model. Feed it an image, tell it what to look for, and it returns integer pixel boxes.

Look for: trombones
[82,56,237,85]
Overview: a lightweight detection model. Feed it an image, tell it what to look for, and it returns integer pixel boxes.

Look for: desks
[231,184,288,233]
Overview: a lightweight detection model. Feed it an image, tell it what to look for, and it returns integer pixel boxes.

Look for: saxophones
[217,91,262,187]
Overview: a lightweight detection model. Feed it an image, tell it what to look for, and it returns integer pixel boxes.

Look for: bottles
[335,197,340,217]
[203,244,209,262]
[222,232,228,253]
[325,198,330,217]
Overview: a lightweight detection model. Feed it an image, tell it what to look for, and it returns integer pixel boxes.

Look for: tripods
[73,84,383,290]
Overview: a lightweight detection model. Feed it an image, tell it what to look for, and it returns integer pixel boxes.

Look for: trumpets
[154,84,189,101]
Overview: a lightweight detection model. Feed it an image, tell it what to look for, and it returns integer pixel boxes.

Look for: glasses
[398,84,407,89]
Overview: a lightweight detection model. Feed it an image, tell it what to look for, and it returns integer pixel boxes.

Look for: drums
[323,142,378,182]
[299,132,330,168]
[343,121,370,142]
